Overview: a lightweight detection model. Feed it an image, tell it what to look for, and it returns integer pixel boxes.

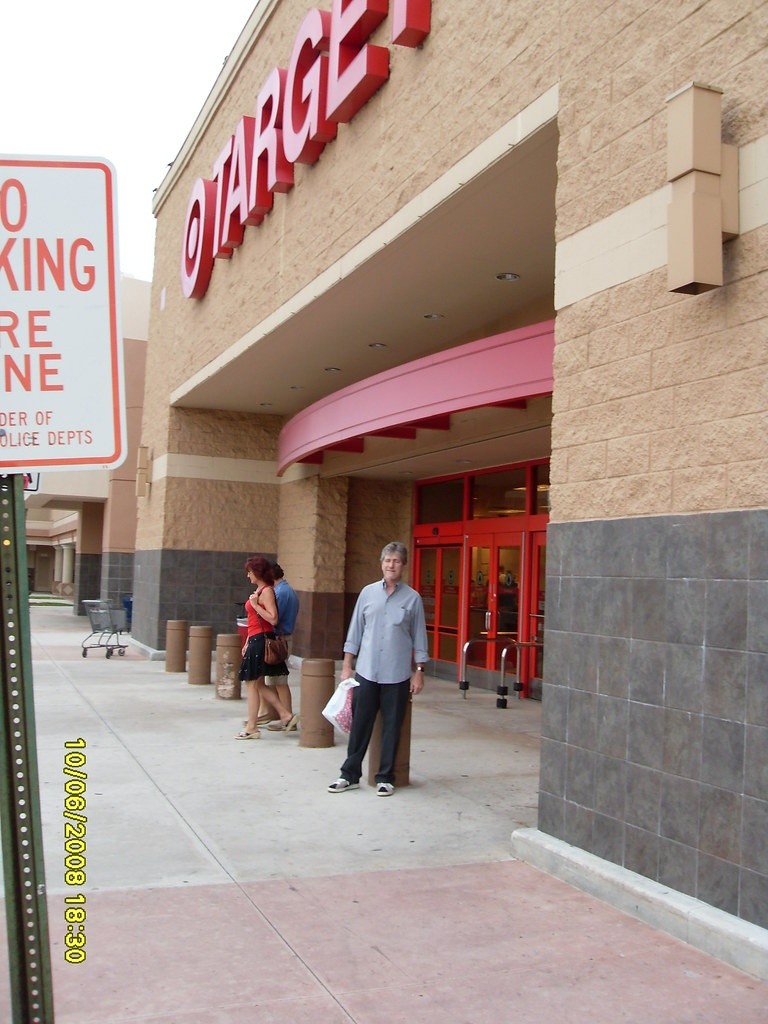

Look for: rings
[250,595,253,598]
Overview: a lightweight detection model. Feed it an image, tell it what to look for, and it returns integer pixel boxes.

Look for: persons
[328,542,431,795]
[232,554,300,741]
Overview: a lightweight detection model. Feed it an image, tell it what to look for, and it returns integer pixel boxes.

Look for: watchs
[415,666,425,671]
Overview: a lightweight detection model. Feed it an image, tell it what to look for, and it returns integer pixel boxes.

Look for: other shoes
[267,723,287,731]
[377,782,395,795]
[328,778,359,792]
[244,714,272,725]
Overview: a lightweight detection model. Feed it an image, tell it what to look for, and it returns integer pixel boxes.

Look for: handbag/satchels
[322,678,360,736]
[264,638,289,664]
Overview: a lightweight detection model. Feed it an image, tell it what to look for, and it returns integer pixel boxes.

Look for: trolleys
[81,600,129,659]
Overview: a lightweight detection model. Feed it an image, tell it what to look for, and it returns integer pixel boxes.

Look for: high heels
[285,713,297,733]
[234,730,261,740]
